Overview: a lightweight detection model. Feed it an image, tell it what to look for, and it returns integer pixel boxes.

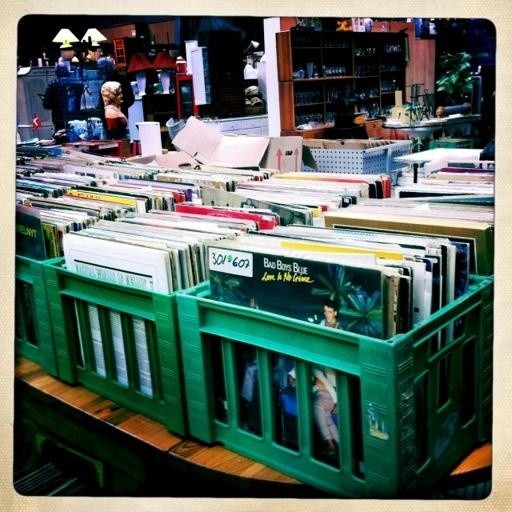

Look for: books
[15,138,495,479]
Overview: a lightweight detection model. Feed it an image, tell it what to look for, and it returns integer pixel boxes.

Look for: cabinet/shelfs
[276,28,407,141]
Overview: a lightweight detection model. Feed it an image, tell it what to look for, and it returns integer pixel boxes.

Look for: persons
[101,81,128,138]
[97,57,135,123]
[43,62,85,132]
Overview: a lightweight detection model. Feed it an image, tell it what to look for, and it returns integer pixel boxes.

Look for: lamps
[52,28,108,49]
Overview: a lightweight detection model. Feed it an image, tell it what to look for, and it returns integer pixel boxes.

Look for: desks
[13,353,492,496]
